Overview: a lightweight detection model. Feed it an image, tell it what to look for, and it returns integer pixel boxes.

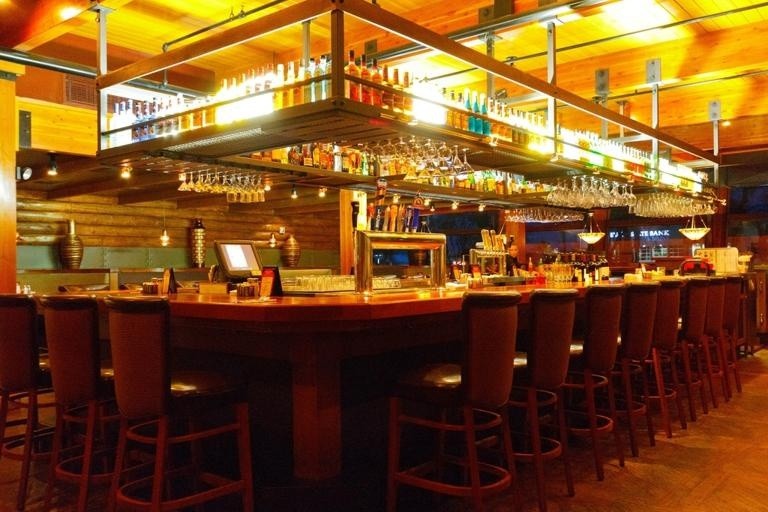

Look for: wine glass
[502,170,714,224]
[175,166,267,193]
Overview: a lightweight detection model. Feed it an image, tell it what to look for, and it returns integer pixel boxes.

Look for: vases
[187,216,207,267]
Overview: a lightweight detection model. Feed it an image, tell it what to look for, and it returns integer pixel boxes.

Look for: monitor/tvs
[213,239,263,278]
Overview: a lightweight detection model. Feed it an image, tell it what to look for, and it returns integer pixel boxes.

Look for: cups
[544,264,574,283]
[291,275,384,290]
[459,273,482,288]
[623,266,665,283]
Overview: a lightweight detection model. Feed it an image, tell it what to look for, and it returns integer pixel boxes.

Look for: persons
[505,244,521,276]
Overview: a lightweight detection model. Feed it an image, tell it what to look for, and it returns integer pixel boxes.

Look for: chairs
[40,294,160,512]
[101,294,257,509]
[0,294,78,512]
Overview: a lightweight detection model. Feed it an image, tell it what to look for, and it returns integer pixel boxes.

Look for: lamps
[577,210,606,245]
[677,215,712,241]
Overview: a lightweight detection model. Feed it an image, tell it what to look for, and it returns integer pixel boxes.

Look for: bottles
[480,229,503,250]
[526,256,533,284]
[365,203,420,233]
[143,281,158,293]
[16,282,30,294]
[568,253,608,284]
[238,140,342,173]
[107,54,331,148]
[236,277,258,296]
[343,48,707,195]
[190,216,207,268]
[535,258,545,285]
